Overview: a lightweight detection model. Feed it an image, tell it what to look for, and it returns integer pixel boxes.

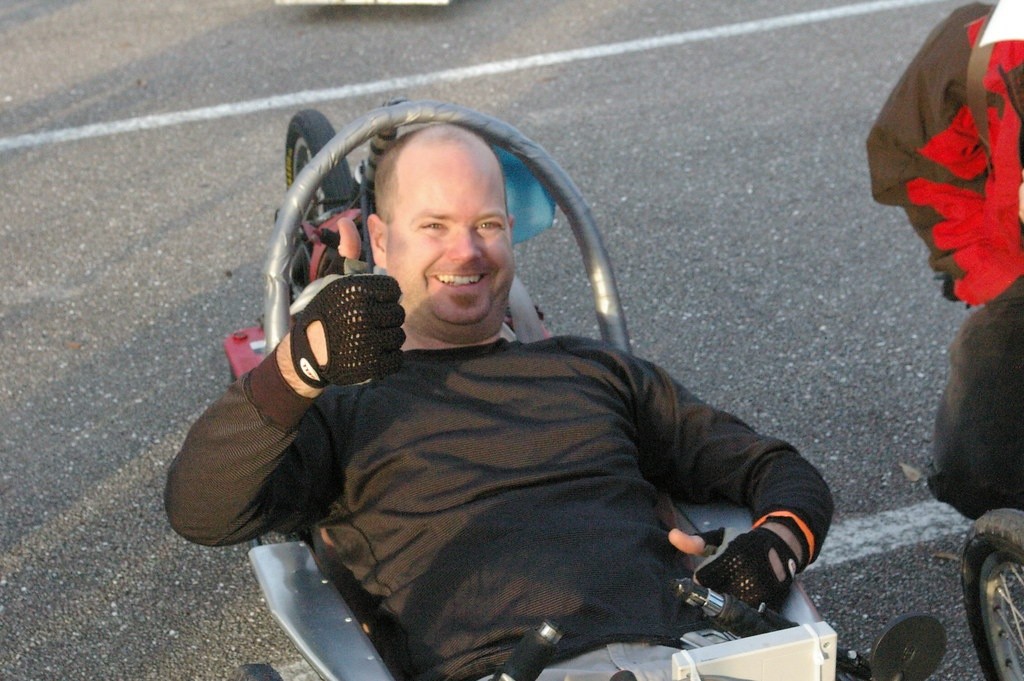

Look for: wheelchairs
[863,0,1024,681]
[162,96,949,681]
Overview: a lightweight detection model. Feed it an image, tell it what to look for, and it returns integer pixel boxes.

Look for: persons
[165,125,834,681]
